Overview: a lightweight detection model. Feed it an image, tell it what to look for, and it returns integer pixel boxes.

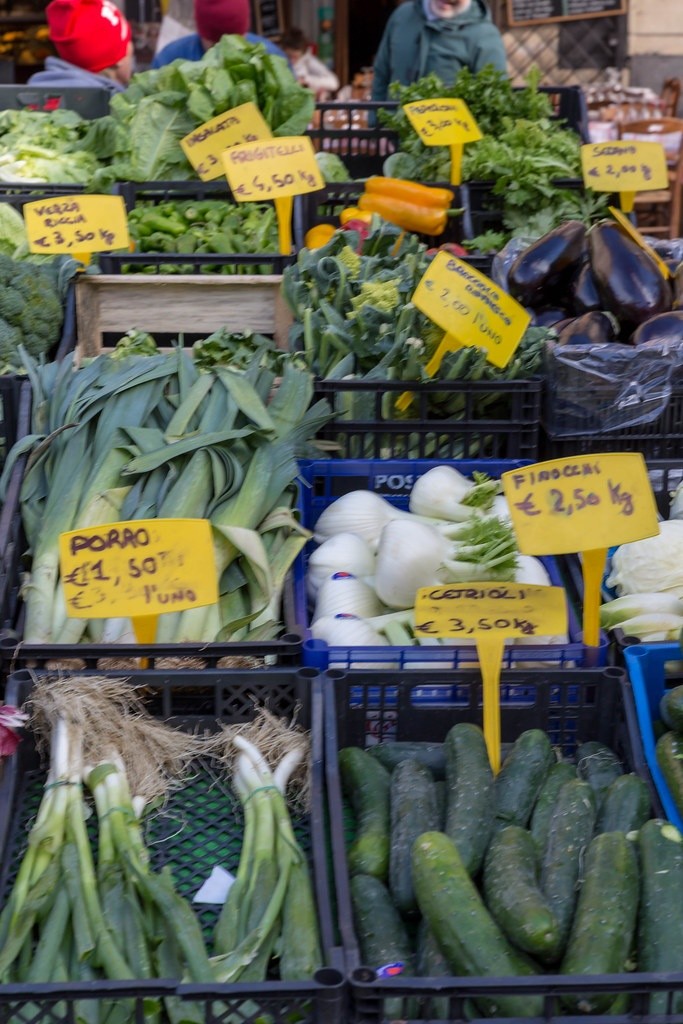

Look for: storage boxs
[462,180,638,276]
[324,666,683,1024]
[300,100,412,242]
[0,382,303,735]
[622,644,683,834]
[91,178,297,275]
[561,458,683,663]
[306,252,543,499]
[295,456,611,705]
[511,83,593,179]
[299,179,488,278]
[52,270,80,365]
[0,668,346,1024]
[0,85,118,195]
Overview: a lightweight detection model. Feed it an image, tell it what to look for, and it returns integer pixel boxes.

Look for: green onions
[0,670,318,1023]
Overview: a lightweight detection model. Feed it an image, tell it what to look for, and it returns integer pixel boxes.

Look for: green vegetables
[377,67,615,255]
[0,35,317,199]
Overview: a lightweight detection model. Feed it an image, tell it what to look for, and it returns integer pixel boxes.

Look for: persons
[278,29,339,91]
[153,0,295,81]
[29,0,135,108]
[366,0,508,128]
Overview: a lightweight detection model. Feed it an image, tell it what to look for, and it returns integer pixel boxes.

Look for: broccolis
[1,198,63,364]
[282,216,546,457]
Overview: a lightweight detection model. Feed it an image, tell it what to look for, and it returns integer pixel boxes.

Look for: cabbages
[594,478,682,643]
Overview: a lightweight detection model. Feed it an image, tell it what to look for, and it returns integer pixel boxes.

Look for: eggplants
[506,219,682,431]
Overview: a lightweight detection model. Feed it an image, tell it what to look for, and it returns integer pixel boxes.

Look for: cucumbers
[337,722,683,1024]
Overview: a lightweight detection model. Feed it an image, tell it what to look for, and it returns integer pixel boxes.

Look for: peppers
[117,199,278,276]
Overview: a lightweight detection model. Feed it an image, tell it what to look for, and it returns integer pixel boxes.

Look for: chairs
[590,85,643,119]
[616,117,683,240]
[312,103,383,155]
[661,77,682,116]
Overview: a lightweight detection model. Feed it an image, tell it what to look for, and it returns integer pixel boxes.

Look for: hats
[194,1,251,43]
[45,0,133,76]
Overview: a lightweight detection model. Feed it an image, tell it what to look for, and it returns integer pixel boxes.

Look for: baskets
[0,78,683,1024]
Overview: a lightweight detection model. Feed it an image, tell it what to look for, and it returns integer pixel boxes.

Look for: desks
[304,103,394,155]
[587,120,683,238]
